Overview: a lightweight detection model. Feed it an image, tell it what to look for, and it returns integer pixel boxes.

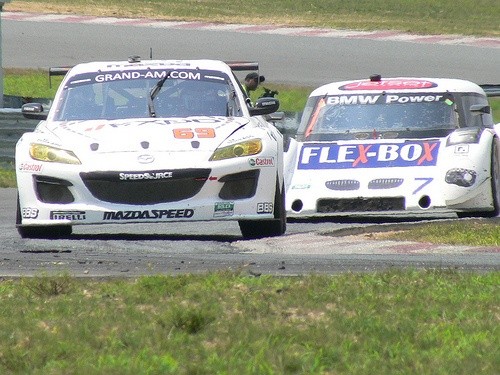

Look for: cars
[13,47,287,239]
[266,72,500,223]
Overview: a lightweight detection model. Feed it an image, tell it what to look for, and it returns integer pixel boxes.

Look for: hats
[245,72,265,82]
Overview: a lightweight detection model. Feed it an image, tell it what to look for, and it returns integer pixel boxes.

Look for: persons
[242,72,265,98]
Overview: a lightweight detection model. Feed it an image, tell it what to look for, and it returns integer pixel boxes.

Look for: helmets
[179,83,218,113]
